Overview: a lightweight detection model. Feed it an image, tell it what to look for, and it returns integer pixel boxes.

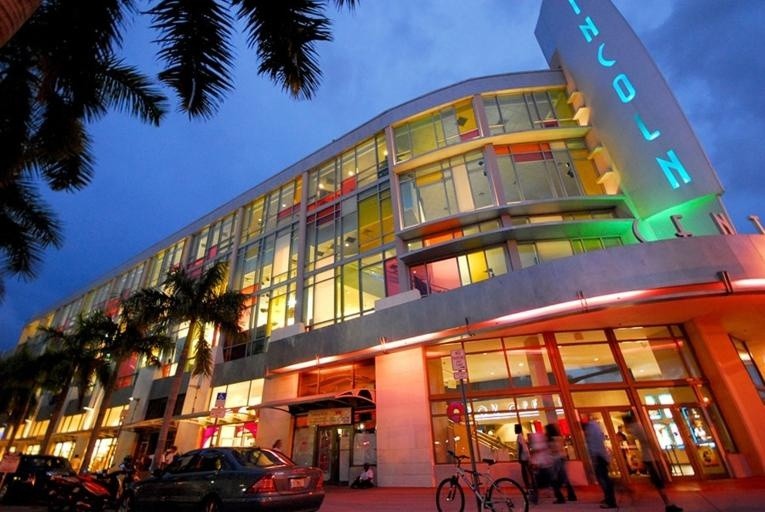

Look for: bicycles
[436,450,529,512]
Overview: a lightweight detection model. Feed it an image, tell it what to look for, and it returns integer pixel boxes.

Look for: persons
[622,409,683,511]
[514,423,577,504]
[271,439,283,453]
[68,454,82,475]
[614,424,638,475]
[579,415,617,509]
[119,447,178,486]
[348,462,375,489]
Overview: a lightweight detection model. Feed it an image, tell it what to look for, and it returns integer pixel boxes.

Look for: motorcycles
[45,461,134,511]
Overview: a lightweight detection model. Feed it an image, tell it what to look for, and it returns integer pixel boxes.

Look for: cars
[0,454,79,506]
[123,446,327,512]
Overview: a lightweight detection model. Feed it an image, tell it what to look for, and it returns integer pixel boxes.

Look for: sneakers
[552,493,577,504]
[529,495,539,505]
[599,499,617,508]
[665,504,683,512]
